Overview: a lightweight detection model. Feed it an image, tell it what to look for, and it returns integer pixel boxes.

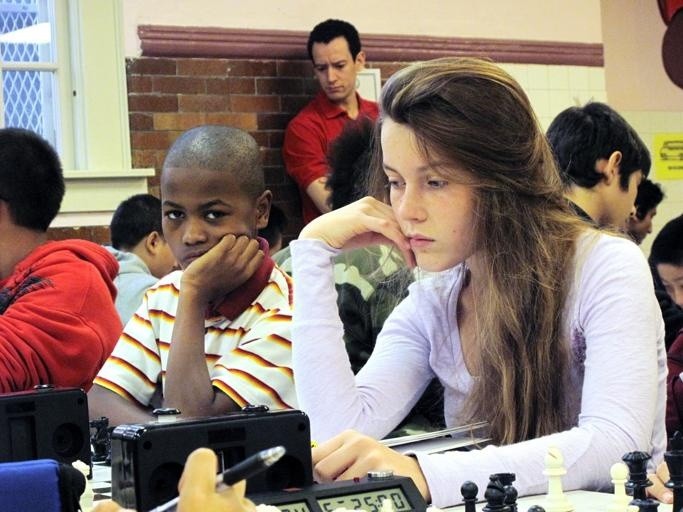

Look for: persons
[542,104,683,505]
[254,202,288,256]
[98,192,177,332]
[277,19,382,230]
[268,116,414,376]
[288,57,670,509]
[1,128,123,396]
[87,124,299,430]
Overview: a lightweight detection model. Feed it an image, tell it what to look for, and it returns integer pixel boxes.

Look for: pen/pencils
[148,445,286,512]
